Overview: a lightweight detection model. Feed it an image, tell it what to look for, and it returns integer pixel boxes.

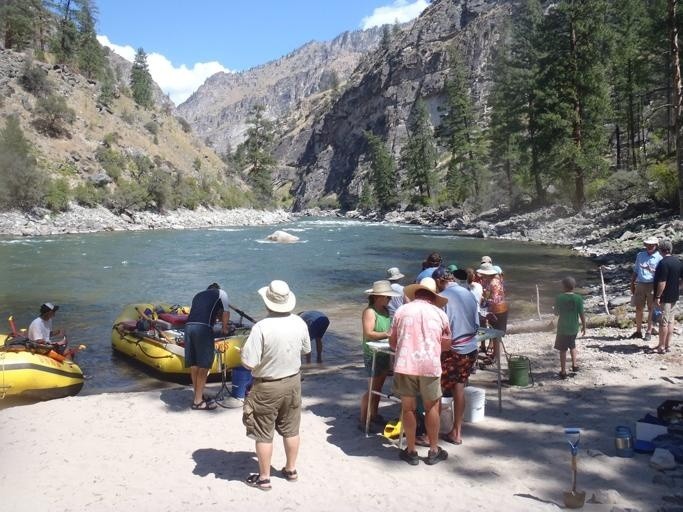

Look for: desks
[364,326,505,454]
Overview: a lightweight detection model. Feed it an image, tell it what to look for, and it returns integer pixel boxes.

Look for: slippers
[439,432,463,446]
[415,435,431,447]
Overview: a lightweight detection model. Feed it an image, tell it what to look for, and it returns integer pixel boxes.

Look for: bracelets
[655,296,660,299]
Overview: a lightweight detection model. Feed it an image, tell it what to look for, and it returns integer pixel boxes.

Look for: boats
[108,301,256,382]
[0,333,85,401]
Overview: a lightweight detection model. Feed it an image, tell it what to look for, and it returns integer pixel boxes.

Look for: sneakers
[571,364,580,371]
[398,447,420,465]
[643,333,652,341]
[358,414,385,433]
[628,331,643,339]
[424,447,448,466]
[559,370,567,380]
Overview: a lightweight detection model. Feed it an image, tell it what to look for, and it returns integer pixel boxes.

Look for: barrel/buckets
[508,353,529,387]
[438,397,454,434]
[231,366,251,399]
[615,426,634,457]
[463,385,485,424]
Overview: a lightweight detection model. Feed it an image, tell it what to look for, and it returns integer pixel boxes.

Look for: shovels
[563,427,585,509]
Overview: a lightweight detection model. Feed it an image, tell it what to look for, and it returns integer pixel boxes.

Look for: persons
[28,303,72,361]
[241,280,311,491]
[630,236,663,340]
[184,283,230,410]
[647,241,683,354]
[553,277,586,379]
[297,310,330,363]
[358,251,508,465]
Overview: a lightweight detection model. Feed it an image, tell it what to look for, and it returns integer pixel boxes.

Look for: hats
[447,263,458,273]
[386,267,406,280]
[643,237,661,248]
[363,280,403,297]
[257,279,297,313]
[39,303,59,317]
[403,277,448,309]
[477,255,503,275]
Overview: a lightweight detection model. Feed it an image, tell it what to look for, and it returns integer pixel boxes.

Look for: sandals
[191,396,218,410]
[245,474,273,491]
[281,466,298,480]
[646,346,673,354]
[477,346,494,355]
